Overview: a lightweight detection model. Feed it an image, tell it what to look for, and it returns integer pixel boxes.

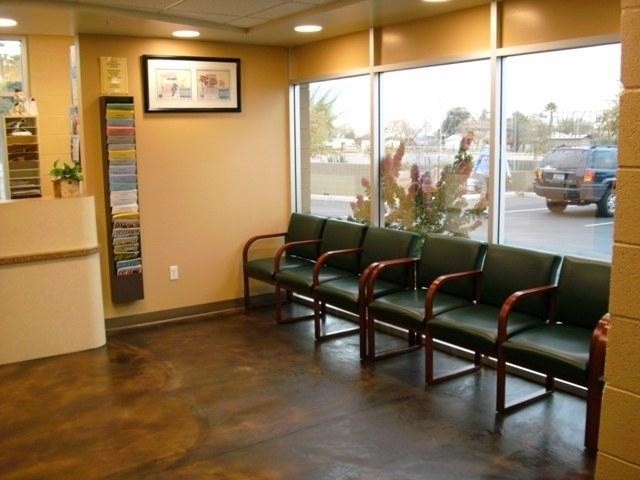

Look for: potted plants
[49,158,84,199]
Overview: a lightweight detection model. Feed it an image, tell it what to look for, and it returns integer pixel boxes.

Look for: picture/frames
[141,55,241,113]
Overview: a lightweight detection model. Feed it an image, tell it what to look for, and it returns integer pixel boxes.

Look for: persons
[473,138,514,207]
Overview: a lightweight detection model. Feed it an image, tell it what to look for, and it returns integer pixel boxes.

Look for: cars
[532,143,618,218]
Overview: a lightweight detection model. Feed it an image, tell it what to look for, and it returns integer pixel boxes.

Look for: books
[104,100,144,278]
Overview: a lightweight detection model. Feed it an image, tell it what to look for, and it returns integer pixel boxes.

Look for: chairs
[366,233,612,449]
[241,211,418,363]
